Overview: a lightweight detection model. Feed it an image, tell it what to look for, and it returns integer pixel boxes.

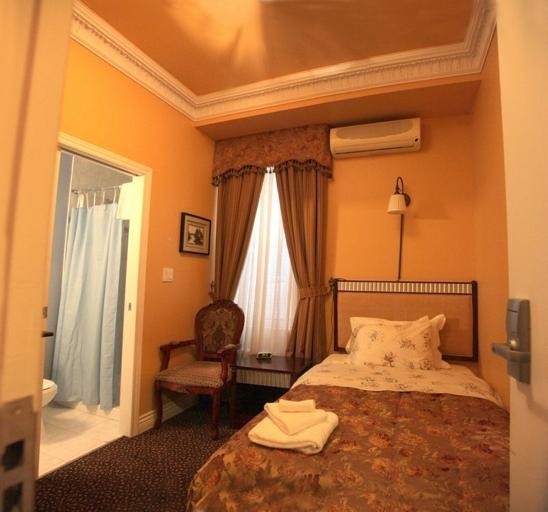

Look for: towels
[248,412,339,454]
[277,399,315,413]
[264,403,326,436]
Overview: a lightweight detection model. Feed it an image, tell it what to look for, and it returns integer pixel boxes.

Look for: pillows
[350,314,451,368]
[346,315,431,354]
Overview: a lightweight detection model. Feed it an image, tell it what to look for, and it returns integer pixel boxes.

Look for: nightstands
[230,356,313,429]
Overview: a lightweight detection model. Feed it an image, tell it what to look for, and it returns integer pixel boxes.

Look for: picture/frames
[180,213,210,254]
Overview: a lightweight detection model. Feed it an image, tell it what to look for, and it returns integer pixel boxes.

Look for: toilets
[40,378,59,441]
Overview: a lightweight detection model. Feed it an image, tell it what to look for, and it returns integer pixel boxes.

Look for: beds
[186,277,511,512]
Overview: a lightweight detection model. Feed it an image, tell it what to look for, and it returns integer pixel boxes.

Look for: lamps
[387,176,411,282]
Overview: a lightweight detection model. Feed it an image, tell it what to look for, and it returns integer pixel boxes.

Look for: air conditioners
[329,117,422,159]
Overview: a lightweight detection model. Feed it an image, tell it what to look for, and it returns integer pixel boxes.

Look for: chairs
[155,298,243,440]
